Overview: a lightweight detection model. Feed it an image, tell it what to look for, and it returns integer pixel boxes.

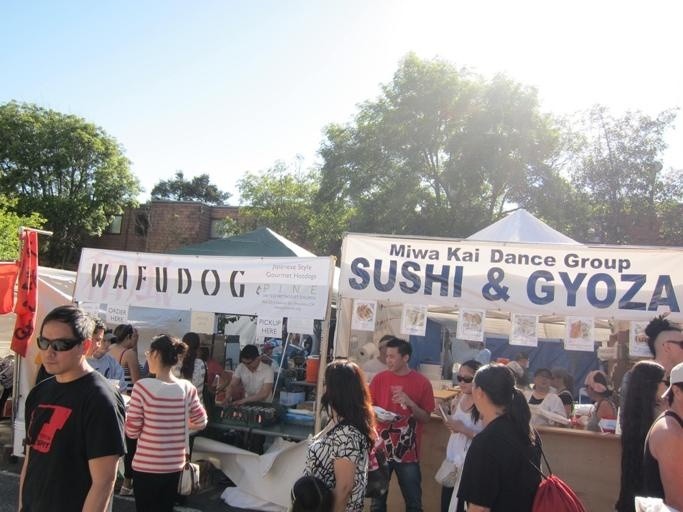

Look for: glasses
[36,336,79,352]
[456,375,473,384]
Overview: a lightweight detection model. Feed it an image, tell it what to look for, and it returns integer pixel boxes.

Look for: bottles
[220,404,275,429]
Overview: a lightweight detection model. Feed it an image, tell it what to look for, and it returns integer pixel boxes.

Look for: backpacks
[524,443,587,511]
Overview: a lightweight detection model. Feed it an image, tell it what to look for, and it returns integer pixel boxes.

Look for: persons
[35,317,223,401]
[632,364,683,511]
[434,343,619,512]
[123,334,208,512]
[364,335,396,384]
[298,358,377,512]
[289,476,334,511]
[367,339,435,512]
[221,345,274,408]
[261,342,272,364]
[17,305,127,512]
[614,361,666,511]
[642,312,683,409]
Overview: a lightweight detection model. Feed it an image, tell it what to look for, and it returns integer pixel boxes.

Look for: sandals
[119,486,136,496]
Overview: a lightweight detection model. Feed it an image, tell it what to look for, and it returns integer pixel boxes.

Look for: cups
[305,353,319,384]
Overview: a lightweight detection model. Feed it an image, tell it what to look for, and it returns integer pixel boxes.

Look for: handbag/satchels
[178,460,201,496]
[435,459,458,488]
[202,387,213,411]
[365,466,392,498]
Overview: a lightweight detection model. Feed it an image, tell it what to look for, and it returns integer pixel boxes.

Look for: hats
[661,362,683,400]
[104,332,117,341]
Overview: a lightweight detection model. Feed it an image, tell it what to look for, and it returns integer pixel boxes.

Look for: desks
[363,413,622,512]
[208,420,312,457]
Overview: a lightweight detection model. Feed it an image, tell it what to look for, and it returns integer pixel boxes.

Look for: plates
[416,363,444,393]
